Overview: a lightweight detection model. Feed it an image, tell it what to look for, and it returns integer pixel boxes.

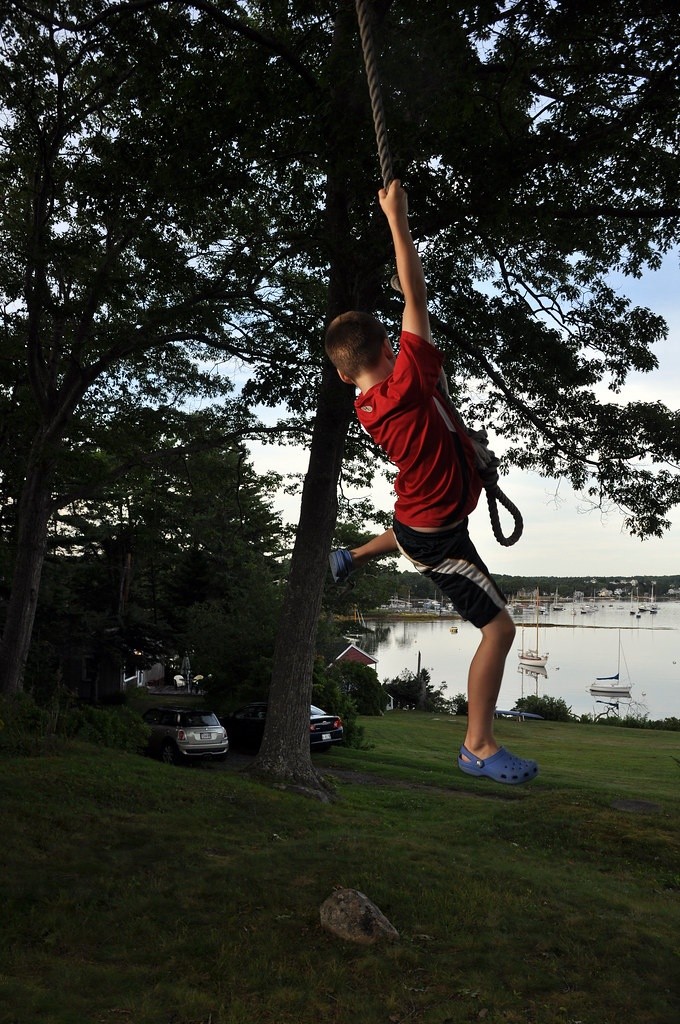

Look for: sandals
[457,743,539,784]
[328,548,355,584]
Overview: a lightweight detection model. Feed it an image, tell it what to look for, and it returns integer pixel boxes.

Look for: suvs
[137,704,230,766]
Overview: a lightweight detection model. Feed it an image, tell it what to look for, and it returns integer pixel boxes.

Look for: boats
[609,604,613,607]
[617,604,624,609]
[503,586,564,615]
[629,583,659,618]
[571,588,604,615]
[449,626,458,633]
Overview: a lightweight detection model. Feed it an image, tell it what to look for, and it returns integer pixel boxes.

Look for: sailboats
[517,663,548,700]
[590,693,632,718]
[518,587,549,667]
[589,627,633,692]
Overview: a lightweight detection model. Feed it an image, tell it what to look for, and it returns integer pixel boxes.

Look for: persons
[324,181,538,783]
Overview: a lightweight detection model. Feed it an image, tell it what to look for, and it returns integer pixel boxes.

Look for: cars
[596,588,623,597]
[389,600,413,608]
[422,600,442,610]
[218,702,344,754]
